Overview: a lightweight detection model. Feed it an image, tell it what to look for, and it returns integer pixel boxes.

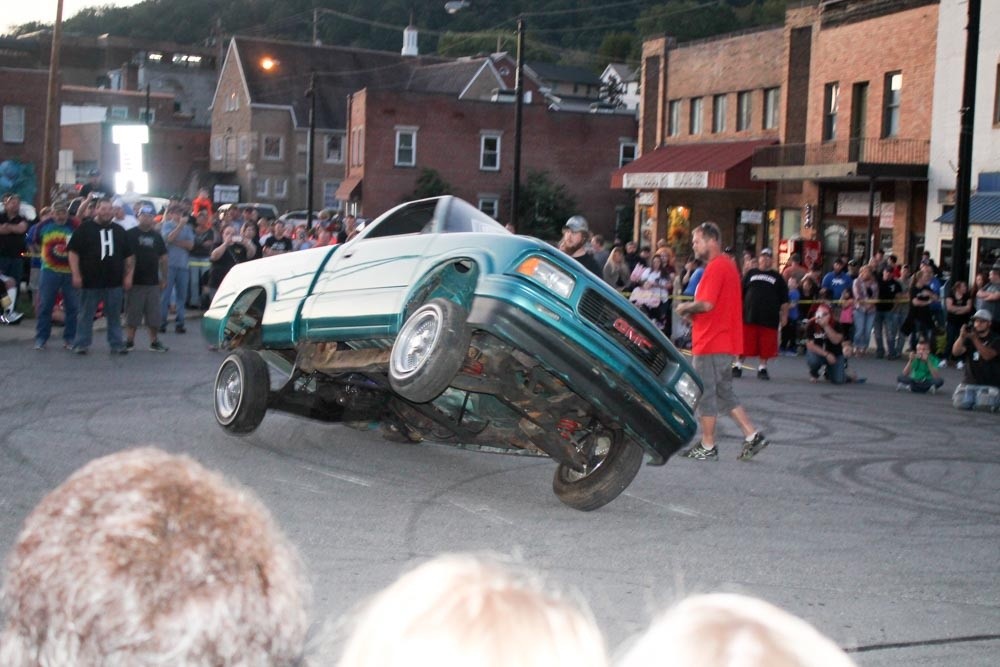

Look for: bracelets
[825,352,830,358]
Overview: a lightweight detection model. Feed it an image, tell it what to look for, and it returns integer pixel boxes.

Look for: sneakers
[737,431,770,462]
[678,443,719,462]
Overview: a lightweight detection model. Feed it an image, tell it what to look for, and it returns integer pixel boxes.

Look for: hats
[135,206,156,219]
[971,309,992,322]
[561,215,589,234]
[51,201,67,212]
[760,248,772,257]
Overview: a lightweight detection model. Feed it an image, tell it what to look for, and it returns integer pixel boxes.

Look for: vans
[215,202,279,223]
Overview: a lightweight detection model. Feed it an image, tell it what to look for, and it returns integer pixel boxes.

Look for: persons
[0,442,862,667]
[0,170,1000,462]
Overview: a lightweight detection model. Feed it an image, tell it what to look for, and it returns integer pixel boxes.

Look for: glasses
[816,310,830,317]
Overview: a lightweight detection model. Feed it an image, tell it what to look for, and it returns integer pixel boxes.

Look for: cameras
[92,192,99,199]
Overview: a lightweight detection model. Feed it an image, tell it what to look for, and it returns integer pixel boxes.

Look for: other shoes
[809,376,817,384]
[895,382,911,393]
[111,343,127,353]
[75,345,88,355]
[3,312,25,325]
[895,352,902,360]
[32,339,45,351]
[159,328,166,333]
[149,341,169,353]
[175,326,187,334]
[758,369,770,381]
[732,366,743,377]
[887,351,895,359]
[939,359,947,368]
[957,361,964,370]
[929,384,936,394]
[62,341,74,351]
[124,340,134,352]
[876,348,885,359]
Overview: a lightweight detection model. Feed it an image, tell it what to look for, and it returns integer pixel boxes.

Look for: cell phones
[966,321,974,333]
[232,236,242,242]
[815,311,822,319]
[915,354,922,358]
[188,216,196,223]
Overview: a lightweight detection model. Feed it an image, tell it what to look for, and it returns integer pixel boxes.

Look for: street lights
[262,57,317,230]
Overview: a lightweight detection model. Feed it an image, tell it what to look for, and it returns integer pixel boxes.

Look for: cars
[201,195,704,512]
[277,209,320,230]
